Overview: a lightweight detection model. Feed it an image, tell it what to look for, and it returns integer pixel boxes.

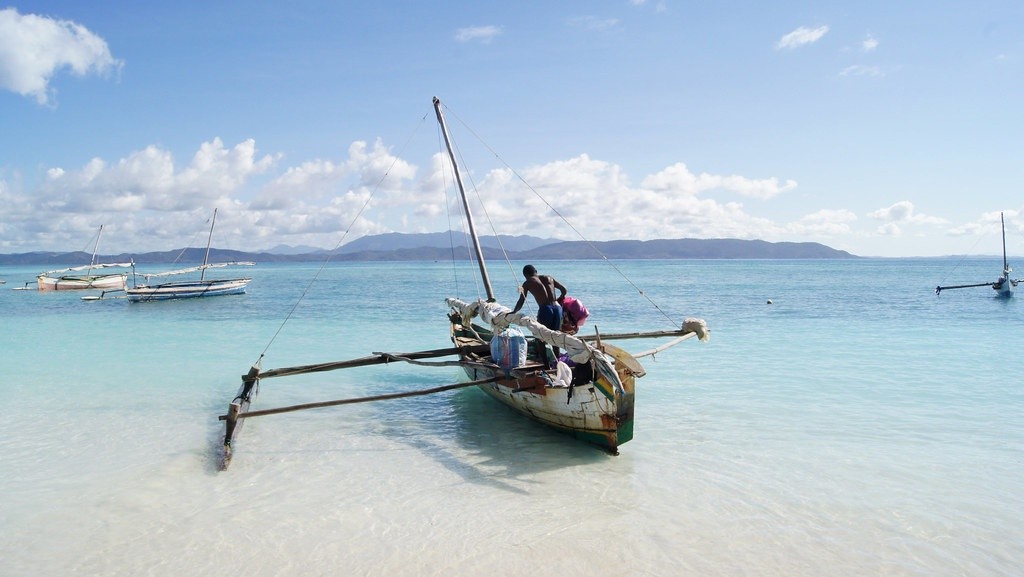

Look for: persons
[504,264,567,375]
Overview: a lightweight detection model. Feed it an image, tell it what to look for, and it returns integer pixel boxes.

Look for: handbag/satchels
[560,296,589,335]
[490,326,528,367]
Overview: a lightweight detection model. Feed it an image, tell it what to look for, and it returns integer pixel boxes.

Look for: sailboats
[994,212,1015,298]
[126,207,251,301]
[14,225,135,289]
[428,95,648,459]
